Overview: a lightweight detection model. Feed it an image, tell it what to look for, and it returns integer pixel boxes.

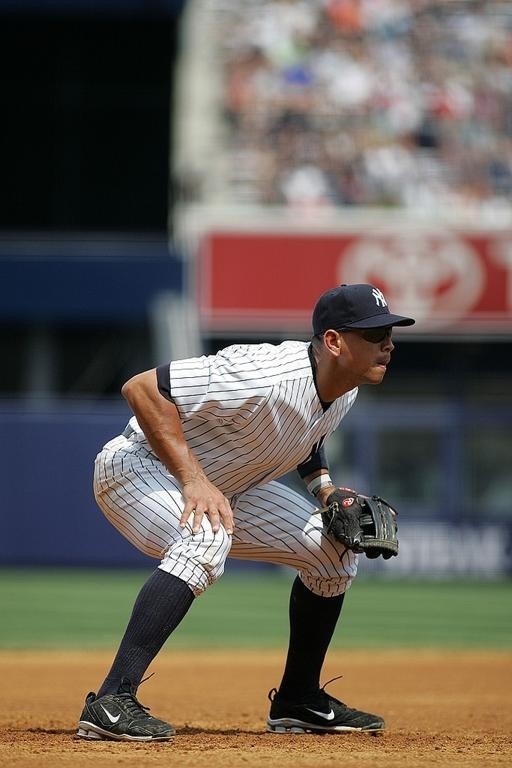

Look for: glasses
[362,327,394,343]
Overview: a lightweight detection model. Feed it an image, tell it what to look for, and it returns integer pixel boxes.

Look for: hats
[313,284,415,338]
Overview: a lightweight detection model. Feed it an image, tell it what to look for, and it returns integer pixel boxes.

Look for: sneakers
[268,676,385,735]
[76,676,178,742]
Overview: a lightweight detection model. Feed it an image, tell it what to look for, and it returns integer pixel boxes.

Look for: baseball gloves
[313,486,399,561]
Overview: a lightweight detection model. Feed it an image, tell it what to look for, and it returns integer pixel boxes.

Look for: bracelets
[311,482,333,497]
[306,473,332,495]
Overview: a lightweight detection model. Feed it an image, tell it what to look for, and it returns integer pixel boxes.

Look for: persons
[214,1,509,213]
[74,279,415,740]
